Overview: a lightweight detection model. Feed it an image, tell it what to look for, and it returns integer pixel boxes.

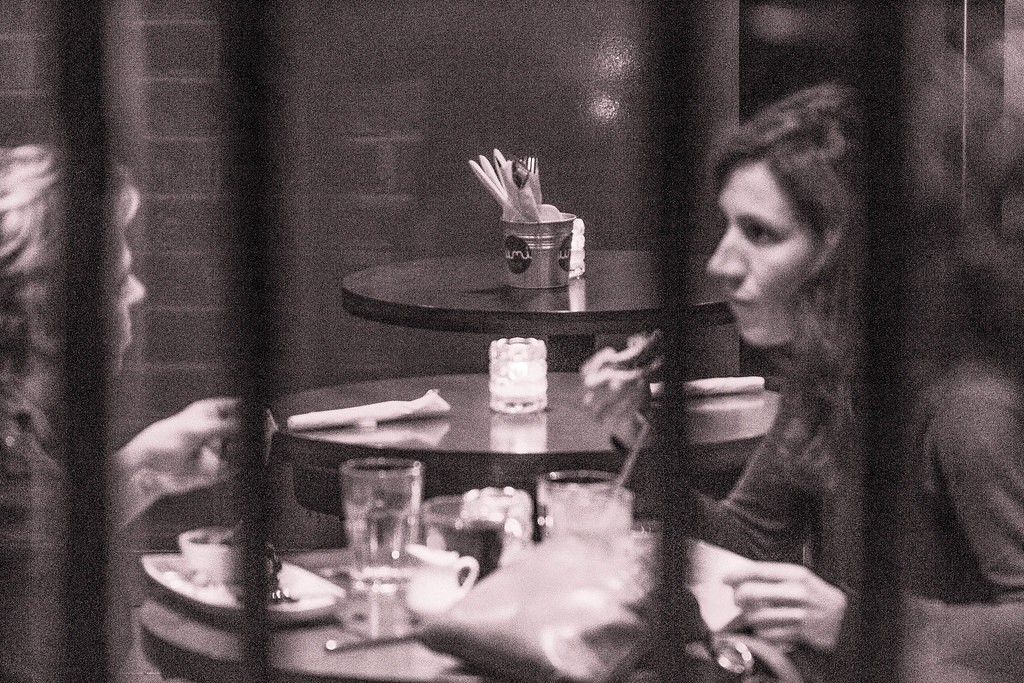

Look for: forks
[510,146,539,222]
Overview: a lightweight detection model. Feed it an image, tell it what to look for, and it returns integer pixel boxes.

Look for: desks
[345,247,733,373]
[269,376,779,516]
[139,543,824,683]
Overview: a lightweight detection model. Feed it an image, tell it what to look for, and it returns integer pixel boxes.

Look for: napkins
[650,375,766,398]
[283,389,447,431]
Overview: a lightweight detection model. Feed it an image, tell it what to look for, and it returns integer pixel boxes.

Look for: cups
[341,459,422,570]
[500,214,575,289]
[491,335,549,414]
[427,498,503,577]
[351,570,409,636]
[463,486,534,548]
[182,529,239,587]
[538,473,632,543]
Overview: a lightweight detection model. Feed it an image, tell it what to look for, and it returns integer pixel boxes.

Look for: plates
[135,552,345,624]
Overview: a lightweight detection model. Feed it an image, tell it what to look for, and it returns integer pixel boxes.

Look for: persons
[0,144,277,683]
[576,82,1024,683]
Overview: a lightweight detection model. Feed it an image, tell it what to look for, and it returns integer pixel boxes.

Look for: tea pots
[404,540,478,616]
